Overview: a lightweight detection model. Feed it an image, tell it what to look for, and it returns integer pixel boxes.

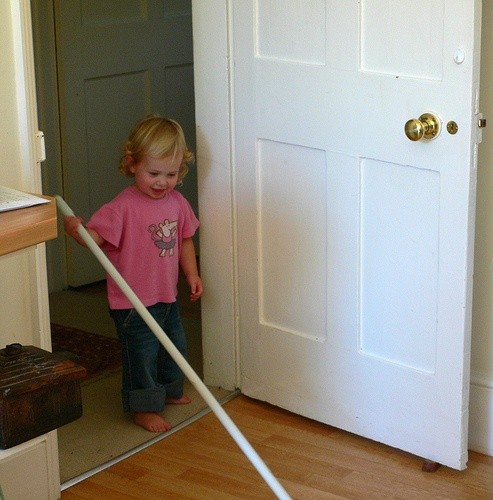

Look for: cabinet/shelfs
[0,185,90,500]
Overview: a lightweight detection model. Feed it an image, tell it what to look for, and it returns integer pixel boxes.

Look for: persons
[62,113,205,432]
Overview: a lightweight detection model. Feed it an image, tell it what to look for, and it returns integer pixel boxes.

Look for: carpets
[49,317,124,391]
